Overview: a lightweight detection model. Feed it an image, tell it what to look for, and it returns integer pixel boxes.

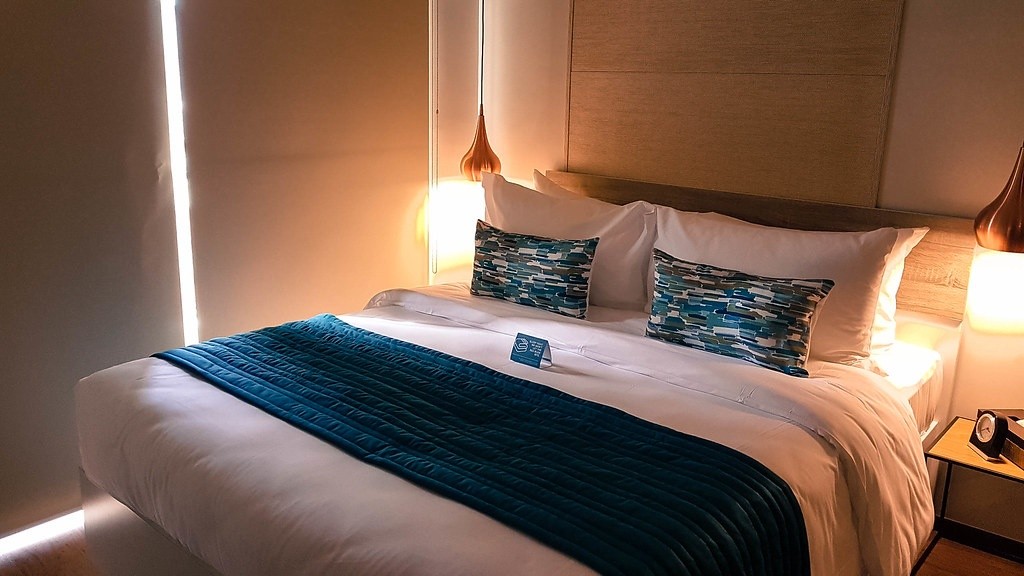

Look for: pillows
[481,169,656,312]
[643,205,930,377]
[469,219,600,321]
[644,248,835,378]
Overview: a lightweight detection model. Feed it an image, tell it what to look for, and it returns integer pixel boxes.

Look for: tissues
[977,408,1024,472]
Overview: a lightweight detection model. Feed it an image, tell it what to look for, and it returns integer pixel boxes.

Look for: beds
[74,169,975,576]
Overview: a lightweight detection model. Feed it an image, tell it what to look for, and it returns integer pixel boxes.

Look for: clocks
[968,410,1008,461]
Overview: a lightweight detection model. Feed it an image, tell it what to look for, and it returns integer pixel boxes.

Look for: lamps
[459,0,501,182]
[974,143,1024,254]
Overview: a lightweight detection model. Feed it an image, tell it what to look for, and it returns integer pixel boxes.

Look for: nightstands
[909,415,1024,576]
